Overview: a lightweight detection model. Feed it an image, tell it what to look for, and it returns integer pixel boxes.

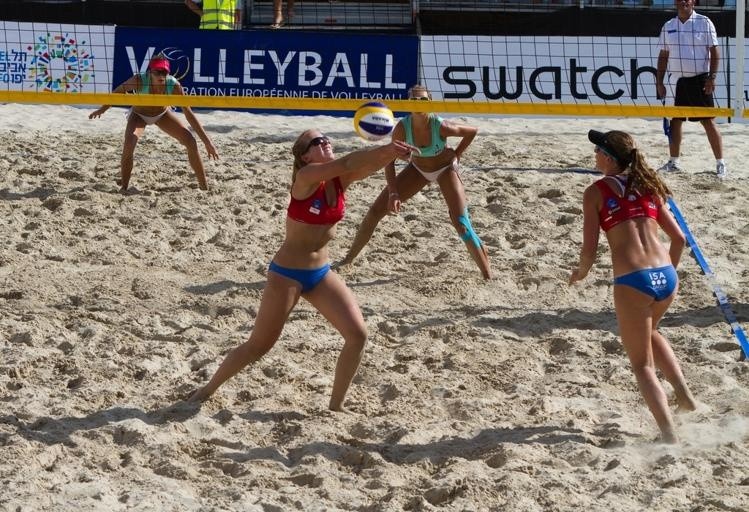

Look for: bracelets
[389,192,399,198]
[709,73,718,80]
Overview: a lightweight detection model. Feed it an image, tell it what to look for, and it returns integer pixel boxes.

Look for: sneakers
[717,162,726,179]
[657,161,682,172]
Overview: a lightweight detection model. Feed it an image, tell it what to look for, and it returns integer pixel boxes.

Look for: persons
[655,0,727,179]
[569,129,698,445]
[88,55,220,194]
[269,0,296,30]
[187,128,422,416]
[183,0,239,30]
[338,83,492,282]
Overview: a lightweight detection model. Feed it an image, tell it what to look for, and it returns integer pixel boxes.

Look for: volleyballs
[354,102,394,140]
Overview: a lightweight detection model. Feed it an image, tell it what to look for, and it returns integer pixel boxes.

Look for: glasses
[306,136,330,150]
[410,96,429,101]
[153,71,168,76]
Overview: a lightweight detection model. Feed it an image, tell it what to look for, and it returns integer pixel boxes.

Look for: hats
[150,59,170,71]
[588,129,621,161]
